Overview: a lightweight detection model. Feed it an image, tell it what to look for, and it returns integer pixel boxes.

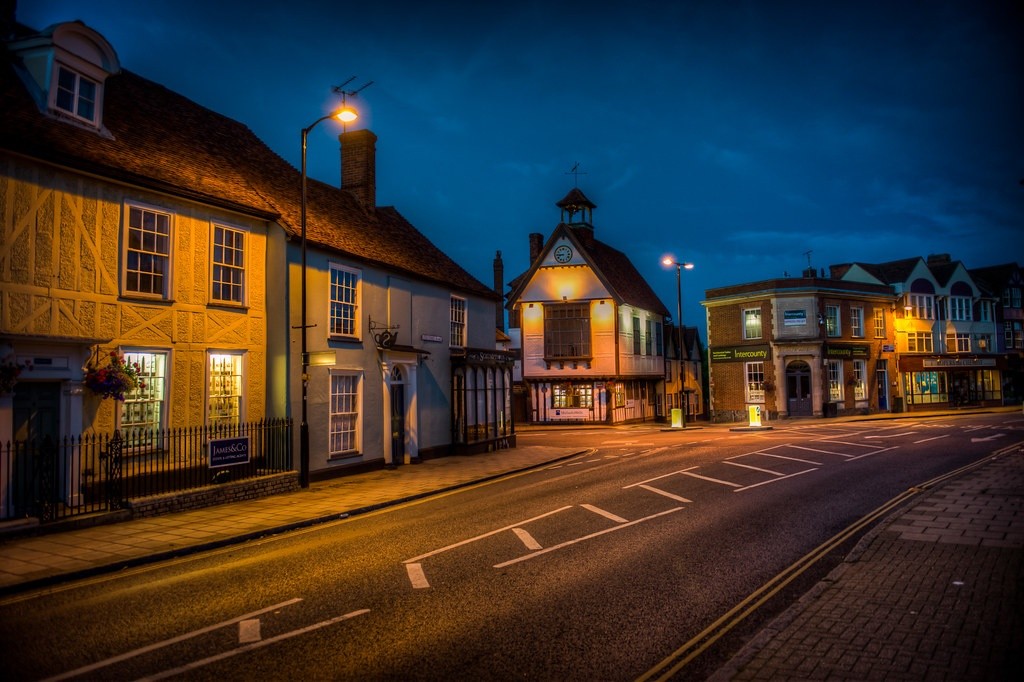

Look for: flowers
[760,378,774,390]
[848,372,859,386]
[80,354,147,403]
[606,381,616,392]
[563,380,572,390]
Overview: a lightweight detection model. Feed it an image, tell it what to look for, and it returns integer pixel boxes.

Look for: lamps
[889,304,914,313]
[528,304,533,308]
[968,355,979,361]
[950,355,960,361]
[600,301,605,305]
[932,355,942,362]
[563,295,568,301]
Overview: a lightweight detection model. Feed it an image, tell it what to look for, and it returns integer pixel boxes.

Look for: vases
[563,384,570,390]
[607,386,615,391]
[849,379,856,385]
[761,385,772,390]
[88,379,131,397]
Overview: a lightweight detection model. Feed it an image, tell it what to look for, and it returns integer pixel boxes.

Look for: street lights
[300,106,359,488]
[661,255,695,430]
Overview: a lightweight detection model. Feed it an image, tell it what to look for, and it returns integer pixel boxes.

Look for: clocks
[554,245,572,263]
